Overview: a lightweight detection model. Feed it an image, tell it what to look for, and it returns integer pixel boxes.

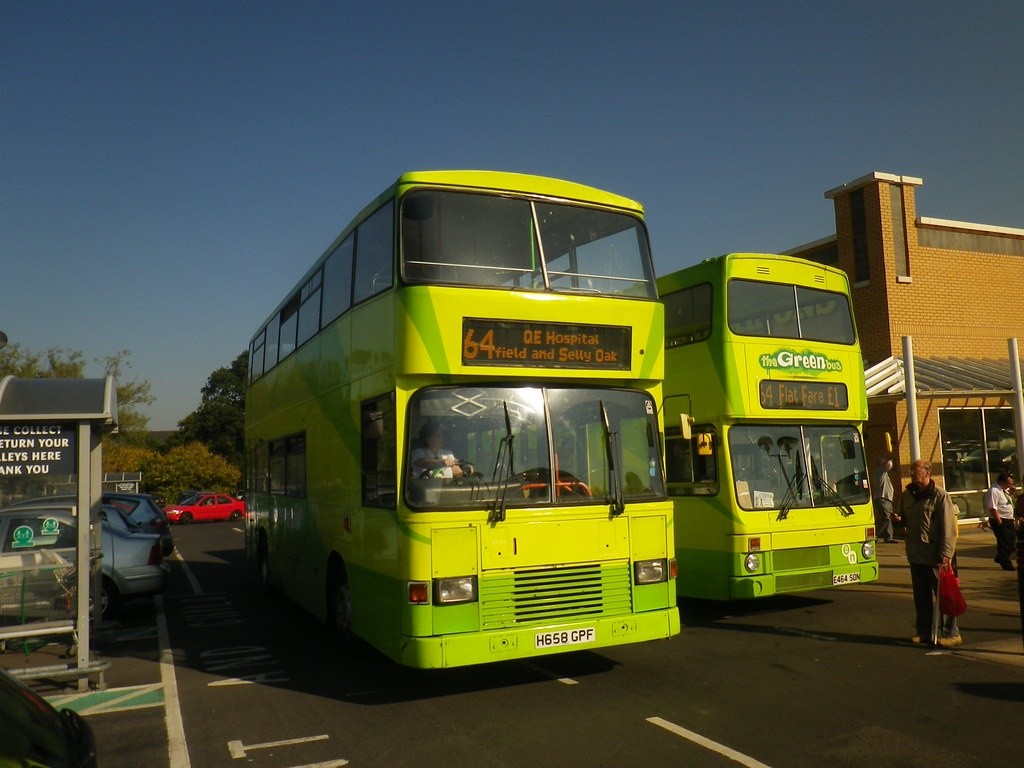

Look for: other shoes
[911,633,935,643]
[884,538,898,543]
[994,559,1016,571]
[938,634,961,647]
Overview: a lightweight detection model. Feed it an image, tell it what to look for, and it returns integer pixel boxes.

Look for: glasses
[908,468,927,474]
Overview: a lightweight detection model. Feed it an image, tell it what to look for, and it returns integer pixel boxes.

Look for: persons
[985,474,1016,571]
[1013,477,1024,526]
[207,498,213,505]
[1007,486,1018,505]
[890,461,963,646]
[410,421,463,479]
[870,456,900,544]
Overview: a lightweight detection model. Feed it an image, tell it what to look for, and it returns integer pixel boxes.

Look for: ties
[1002,489,1012,506]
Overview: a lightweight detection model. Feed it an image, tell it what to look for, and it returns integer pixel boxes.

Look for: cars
[0,490,176,625]
[161,493,247,525]
[959,448,1012,473]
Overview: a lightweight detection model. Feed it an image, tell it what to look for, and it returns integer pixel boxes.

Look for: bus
[245,168,682,670]
[466,251,882,603]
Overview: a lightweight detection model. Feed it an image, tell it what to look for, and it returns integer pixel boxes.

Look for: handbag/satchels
[939,562,967,616]
